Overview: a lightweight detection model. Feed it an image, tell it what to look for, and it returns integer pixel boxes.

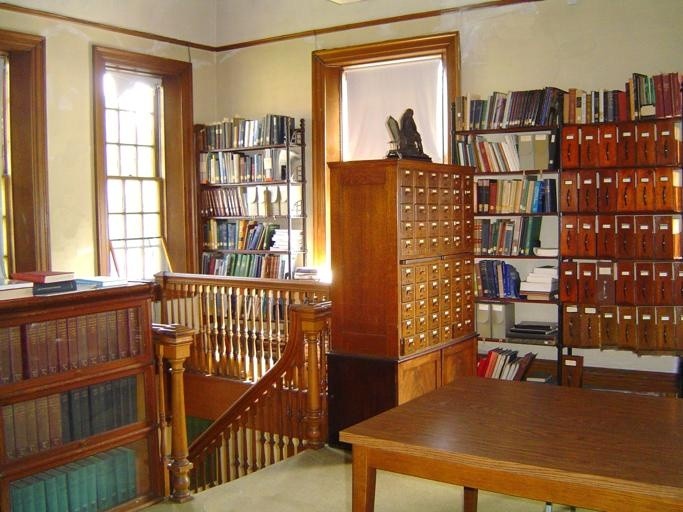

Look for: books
[74,275,129,287]
[198,113,319,322]
[0,305,145,512]
[0,279,35,300]
[33,280,78,295]
[561,71,682,386]
[455,87,561,384]
[9,270,76,284]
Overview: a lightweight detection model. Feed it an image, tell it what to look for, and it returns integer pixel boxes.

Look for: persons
[401,108,424,153]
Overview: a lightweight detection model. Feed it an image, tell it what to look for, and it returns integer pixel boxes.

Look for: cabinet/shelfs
[452,89,565,389]
[328,159,473,450]
[559,110,683,373]
[195,117,306,356]
[1,278,158,512]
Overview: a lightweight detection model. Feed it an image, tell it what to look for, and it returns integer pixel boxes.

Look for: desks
[339,374,682,510]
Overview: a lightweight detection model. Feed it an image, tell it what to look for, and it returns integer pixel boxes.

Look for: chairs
[563,354,583,389]
